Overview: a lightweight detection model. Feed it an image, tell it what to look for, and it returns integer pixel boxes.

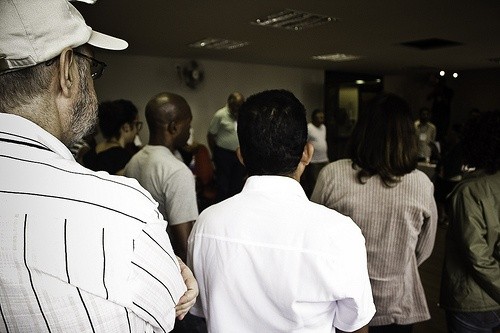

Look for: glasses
[46,50,109,81]
[131,121,143,132]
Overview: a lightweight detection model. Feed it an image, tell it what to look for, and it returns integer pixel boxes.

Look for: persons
[443,108,500,333]
[413,107,436,163]
[309,92,438,333]
[78,98,139,176]
[0,0,199,333]
[124,92,199,265]
[207,91,245,203]
[186,90,375,333]
[307,109,329,193]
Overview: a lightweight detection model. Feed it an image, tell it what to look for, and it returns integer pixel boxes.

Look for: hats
[0,0,129,76]
